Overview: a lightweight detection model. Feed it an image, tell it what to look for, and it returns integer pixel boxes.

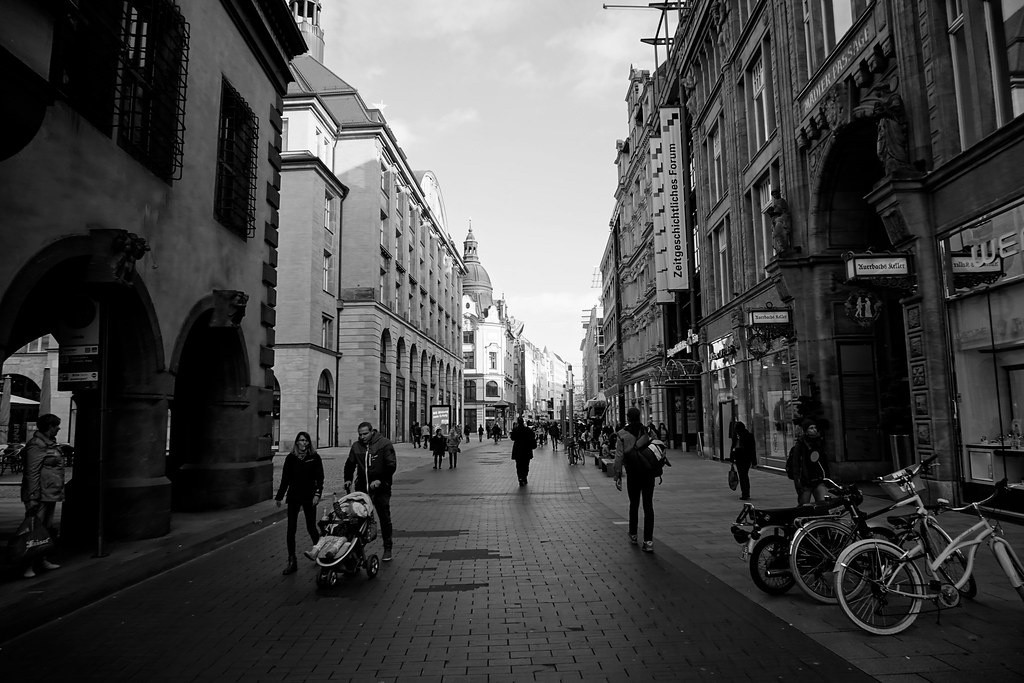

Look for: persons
[431,429,447,469]
[526,420,591,451]
[730,421,756,504]
[601,420,668,459]
[478,424,484,442]
[784,419,829,506]
[446,429,460,469]
[510,418,538,487]
[486,423,502,443]
[275,431,324,575]
[613,407,656,551]
[343,422,397,561]
[410,421,431,449]
[767,189,793,254]
[452,420,463,440]
[21,414,65,578]
[464,423,471,443]
[872,82,909,174]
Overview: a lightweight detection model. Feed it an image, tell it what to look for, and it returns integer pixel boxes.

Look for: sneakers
[382,549,392,561]
[628,533,638,543]
[642,541,654,551]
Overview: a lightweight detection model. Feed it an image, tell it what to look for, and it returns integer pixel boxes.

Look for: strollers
[303,484,385,597]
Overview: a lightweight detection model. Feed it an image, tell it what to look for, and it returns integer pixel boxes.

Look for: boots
[325,547,337,559]
[304,548,319,561]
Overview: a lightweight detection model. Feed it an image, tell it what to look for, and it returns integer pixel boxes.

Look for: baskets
[879,464,925,501]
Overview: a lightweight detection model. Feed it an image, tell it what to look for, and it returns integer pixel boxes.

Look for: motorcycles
[729,446,900,597]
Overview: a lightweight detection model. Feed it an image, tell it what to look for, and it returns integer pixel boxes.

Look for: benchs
[601,458,616,477]
[592,452,604,469]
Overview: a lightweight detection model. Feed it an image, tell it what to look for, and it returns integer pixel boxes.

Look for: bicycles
[787,454,979,605]
[0,442,25,475]
[830,474,1024,637]
[565,439,586,465]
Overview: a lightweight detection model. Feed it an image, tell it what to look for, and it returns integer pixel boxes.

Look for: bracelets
[315,494,320,497]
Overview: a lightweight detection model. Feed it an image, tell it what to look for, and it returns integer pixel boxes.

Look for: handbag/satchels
[7,515,53,566]
[785,446,800,480]
[728,463,738,491]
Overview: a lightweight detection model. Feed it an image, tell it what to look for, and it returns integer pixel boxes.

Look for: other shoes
[42,559,60,569]
[413,444,421,448]
[24,567,35,577]
[740,496,748,500]
[519,479,527,487]
[283,560,297,575]
[432,465,456,469]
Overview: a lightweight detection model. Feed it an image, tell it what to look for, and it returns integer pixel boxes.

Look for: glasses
[299,439,308,442]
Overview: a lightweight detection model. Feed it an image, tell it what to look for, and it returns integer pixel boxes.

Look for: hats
[36,414,60,434]
[801,419,815,432]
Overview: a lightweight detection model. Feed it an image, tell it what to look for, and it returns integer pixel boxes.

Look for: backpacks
[632,427,671,485]
